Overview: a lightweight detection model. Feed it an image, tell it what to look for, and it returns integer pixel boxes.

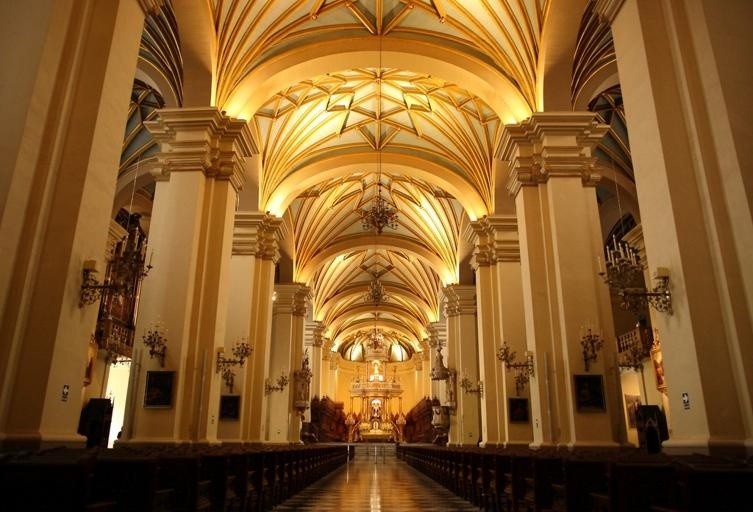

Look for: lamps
[215,333,254,367]
[579,318,604,374]
[461,374,483,396]
[137,319,168,367]
[362,276,390,308]
[266,371,291,393]
[77,226,155,308]
[362,191,400,233]
[489,343,535,375]
[357,328,402,352]
[597,230,675,316]
